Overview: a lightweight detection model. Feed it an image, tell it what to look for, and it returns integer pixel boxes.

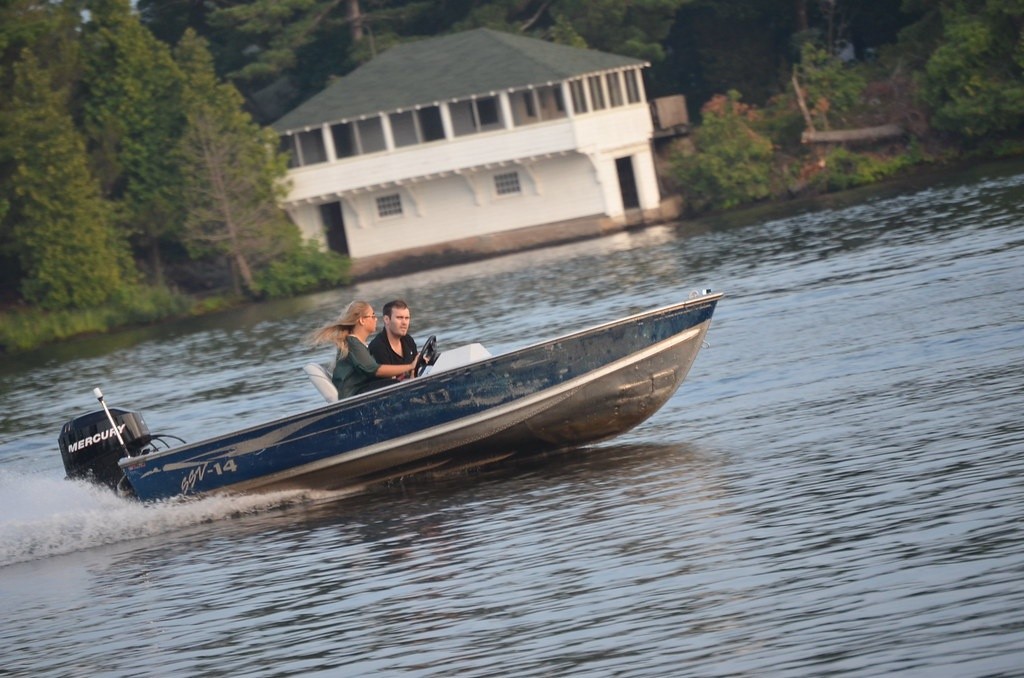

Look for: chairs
[303,361,339,405]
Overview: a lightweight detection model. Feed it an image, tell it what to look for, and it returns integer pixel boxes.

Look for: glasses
[362,313,377,320]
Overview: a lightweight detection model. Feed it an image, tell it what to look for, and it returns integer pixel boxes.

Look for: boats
[54,287,725,507]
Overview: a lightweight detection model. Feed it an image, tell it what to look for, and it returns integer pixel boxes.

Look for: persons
[368,301,418,381]
[305,300,429,401]
